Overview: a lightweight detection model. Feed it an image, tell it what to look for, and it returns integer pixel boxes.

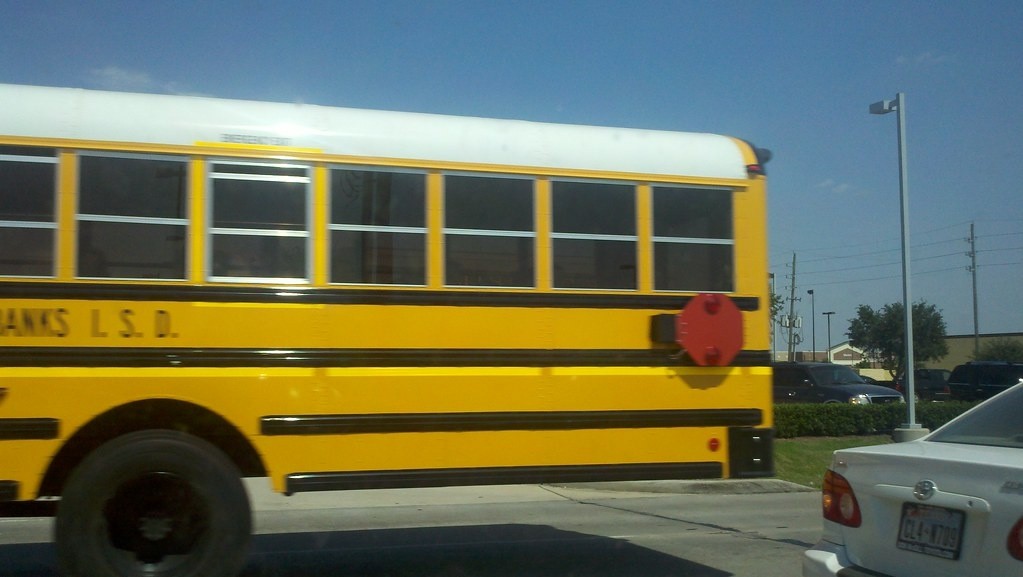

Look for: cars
[802,379,1022,577]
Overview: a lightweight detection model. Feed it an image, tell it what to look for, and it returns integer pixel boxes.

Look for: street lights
[823,311,836,361]
[768,273,775,362]
[867,92,919,427]
[808,288,817,361]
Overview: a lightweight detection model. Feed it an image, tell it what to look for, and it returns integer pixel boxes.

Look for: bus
[1,82,775,577]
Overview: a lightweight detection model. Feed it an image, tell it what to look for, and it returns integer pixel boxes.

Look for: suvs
[893,368,953,403]
[943,359,1023,400]
[773,361,905,406]
